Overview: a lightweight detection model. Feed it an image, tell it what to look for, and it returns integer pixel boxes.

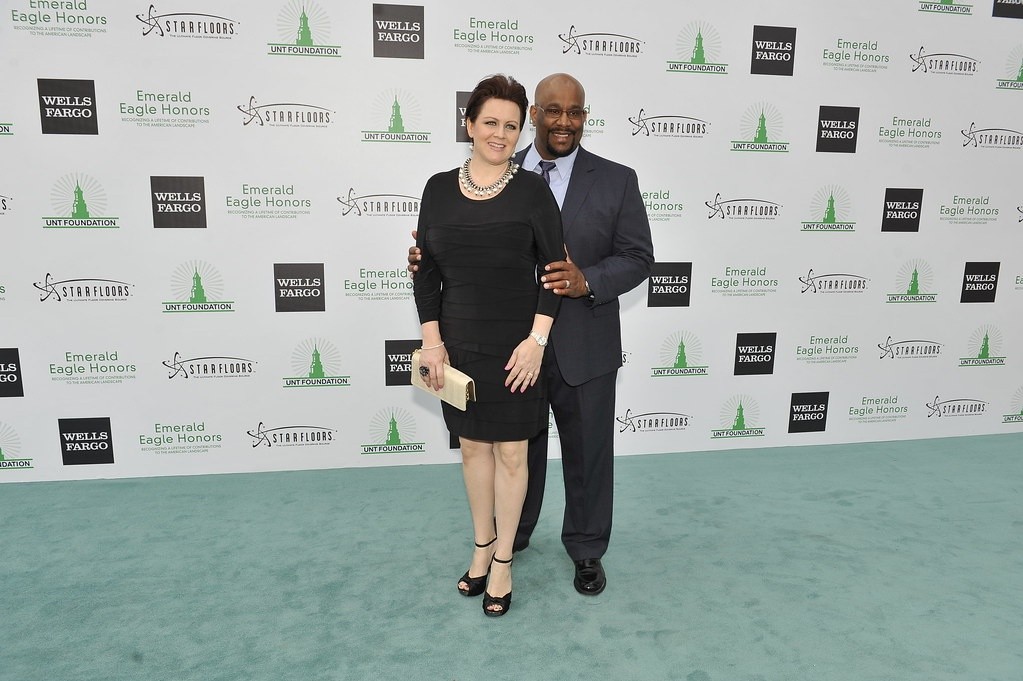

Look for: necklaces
[459,154,520,197]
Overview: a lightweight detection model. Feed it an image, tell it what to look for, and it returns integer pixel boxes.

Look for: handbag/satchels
[410,349,476,410]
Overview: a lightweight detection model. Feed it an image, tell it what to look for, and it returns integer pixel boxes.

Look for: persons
[413,73,570,619]
[407,69,656,598]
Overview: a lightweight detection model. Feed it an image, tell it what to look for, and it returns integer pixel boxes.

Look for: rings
[566,279,571,289]
[526,371,534,378]
[418,364,431,377]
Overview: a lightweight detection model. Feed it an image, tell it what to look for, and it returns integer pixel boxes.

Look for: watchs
[585,271,594,297]
[528,330,549,348]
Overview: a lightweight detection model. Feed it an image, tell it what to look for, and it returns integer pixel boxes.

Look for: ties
[538,160,557,189]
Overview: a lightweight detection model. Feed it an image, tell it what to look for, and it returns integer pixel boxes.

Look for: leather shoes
[513,539,529,555]
[572,557,607,596]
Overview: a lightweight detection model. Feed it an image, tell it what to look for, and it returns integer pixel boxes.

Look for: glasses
[534,104,586,122]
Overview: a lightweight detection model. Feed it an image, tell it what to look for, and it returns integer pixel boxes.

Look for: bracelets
[420,339,446,351]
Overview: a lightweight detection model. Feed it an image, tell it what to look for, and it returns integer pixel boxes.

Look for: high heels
[481,553,512,617]
[458,535,497,596]
